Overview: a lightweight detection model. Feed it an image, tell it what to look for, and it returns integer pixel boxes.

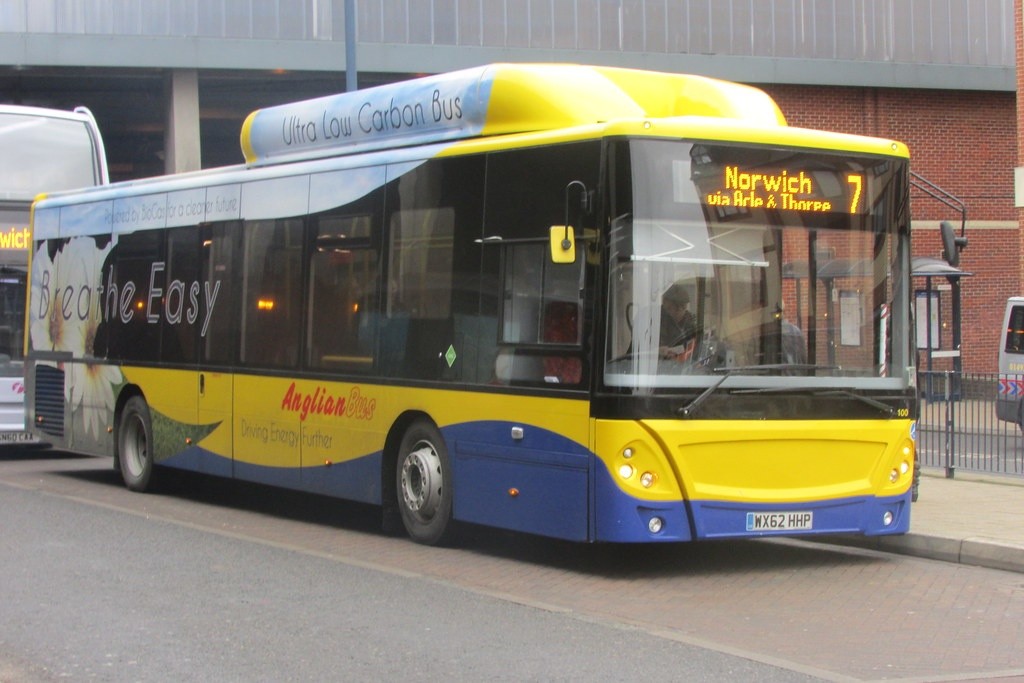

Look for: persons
[625,284,697,363]
[748,297,808,376]
[542,309,585,386]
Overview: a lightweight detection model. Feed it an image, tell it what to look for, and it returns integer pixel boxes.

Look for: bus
[1,99,110,448]
[23,61,969,552]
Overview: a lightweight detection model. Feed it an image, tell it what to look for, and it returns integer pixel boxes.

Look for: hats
[768,299,785,312]
[662,285,689,304]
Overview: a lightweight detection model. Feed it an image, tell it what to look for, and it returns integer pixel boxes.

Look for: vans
[995,296,1024,438]
